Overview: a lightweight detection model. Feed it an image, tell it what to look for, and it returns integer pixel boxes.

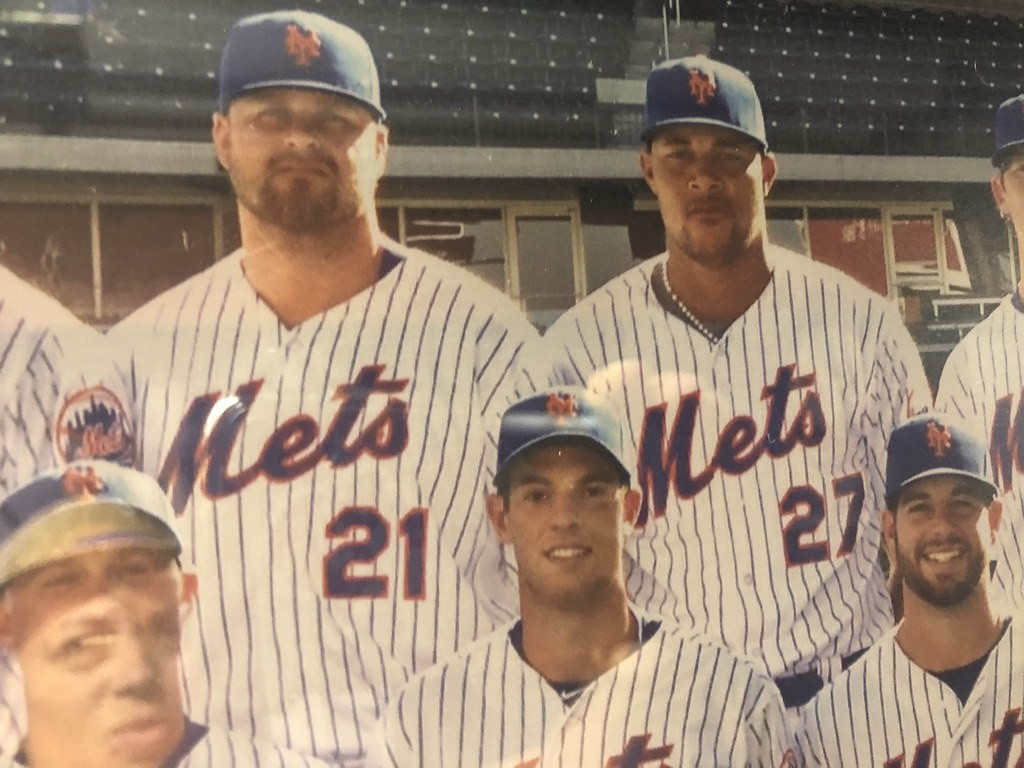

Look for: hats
[0,459,184,585]
[641,53,768,158]
[991,94,1024,165]
[218,11,388,121]
[886,413,1000,498]
[492,386,632,490]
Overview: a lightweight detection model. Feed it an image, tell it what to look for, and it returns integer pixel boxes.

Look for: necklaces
[661,258,718,345]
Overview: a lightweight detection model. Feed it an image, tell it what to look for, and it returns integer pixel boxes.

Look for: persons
[0,11,1024,768]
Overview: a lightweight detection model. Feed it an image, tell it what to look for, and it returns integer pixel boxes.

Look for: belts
[776,645,869,708]
